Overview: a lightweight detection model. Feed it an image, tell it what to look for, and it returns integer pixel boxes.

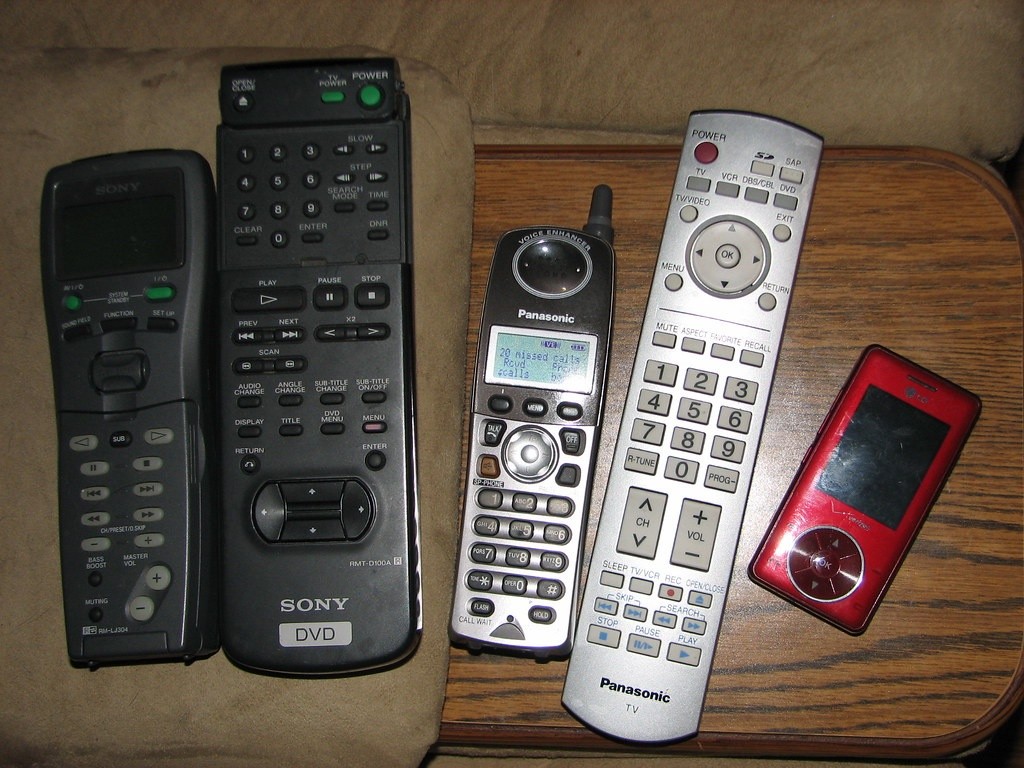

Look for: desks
[430,149,1024,768]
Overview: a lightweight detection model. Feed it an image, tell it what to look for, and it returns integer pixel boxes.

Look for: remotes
[212,53,425,682]
[559,106,825,745]
[40,149,224,673]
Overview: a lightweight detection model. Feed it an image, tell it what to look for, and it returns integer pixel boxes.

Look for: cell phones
[447,183,617,664]
[746,343,981,638]
